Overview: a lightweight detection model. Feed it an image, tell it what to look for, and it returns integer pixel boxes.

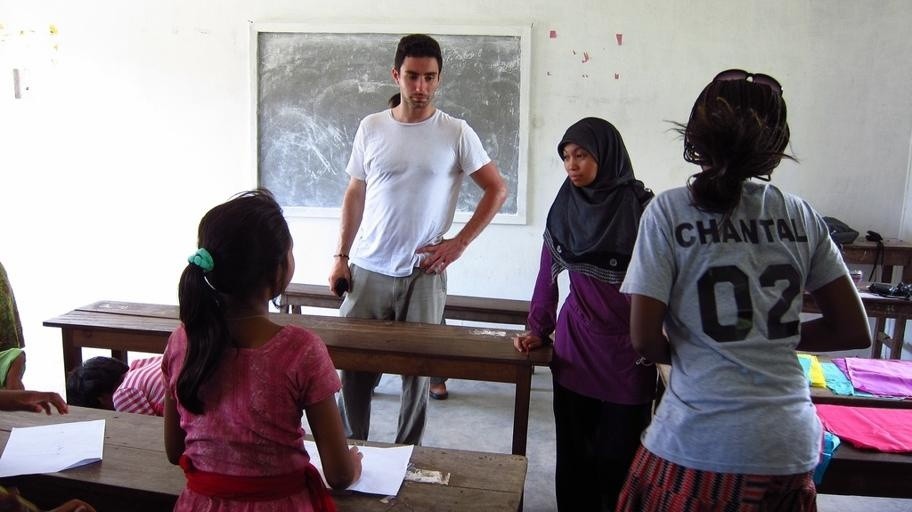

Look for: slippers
[429,387,448,399]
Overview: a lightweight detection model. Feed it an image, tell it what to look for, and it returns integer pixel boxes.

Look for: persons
[65,355,164,418]
[161,190,363,511]
[387,94,449,400]
[513,117,656,511]
[327,35,506,444]
[617,69,871,511]
[0,348,93,512]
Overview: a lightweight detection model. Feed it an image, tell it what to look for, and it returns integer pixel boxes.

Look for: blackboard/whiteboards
[247,19,532,226]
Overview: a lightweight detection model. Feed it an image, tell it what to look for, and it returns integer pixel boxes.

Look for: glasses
[711,68,784,94]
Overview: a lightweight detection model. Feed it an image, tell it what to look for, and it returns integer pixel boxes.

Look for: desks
[41,299,554,511]
[656,352,911,407]
[838,234,912,284]
[801,286,912,360]
[814,403,912,499]
[0,402,529,512]
[276,282,531,329]
